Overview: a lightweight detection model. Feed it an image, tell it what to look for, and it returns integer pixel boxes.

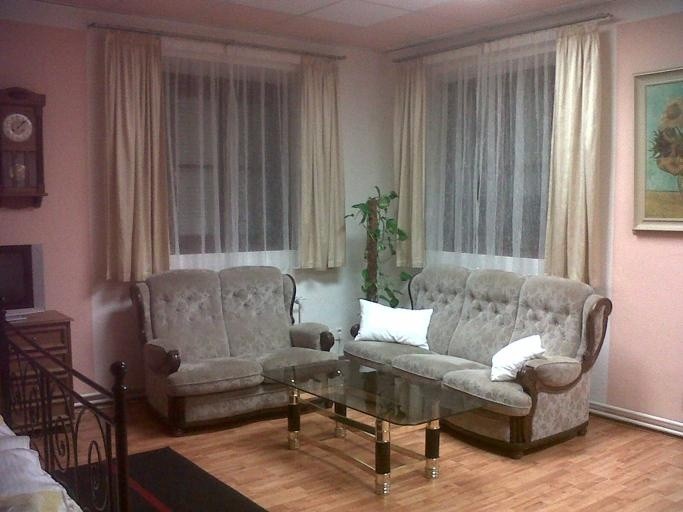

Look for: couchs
[131,264,333,437]
[344,263,612,459]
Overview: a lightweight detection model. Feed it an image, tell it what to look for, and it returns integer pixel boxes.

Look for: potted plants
[343,185,413,338]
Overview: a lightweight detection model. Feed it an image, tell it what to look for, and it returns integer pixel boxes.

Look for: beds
[0,313,129,512]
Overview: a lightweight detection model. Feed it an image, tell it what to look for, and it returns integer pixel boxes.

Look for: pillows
[355,298,433,351]
[491,335,546,382]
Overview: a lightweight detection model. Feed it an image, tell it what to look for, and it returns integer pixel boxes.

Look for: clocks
[1,86,49,209]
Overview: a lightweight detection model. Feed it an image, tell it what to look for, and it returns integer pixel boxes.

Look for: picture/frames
[632,66,683,237]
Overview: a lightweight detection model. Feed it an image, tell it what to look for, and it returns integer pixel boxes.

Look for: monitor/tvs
[1,242,46,322]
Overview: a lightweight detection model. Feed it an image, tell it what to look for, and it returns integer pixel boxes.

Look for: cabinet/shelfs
[8,311,74,436]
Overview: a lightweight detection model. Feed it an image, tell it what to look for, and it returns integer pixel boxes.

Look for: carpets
[48,447,268,511]
